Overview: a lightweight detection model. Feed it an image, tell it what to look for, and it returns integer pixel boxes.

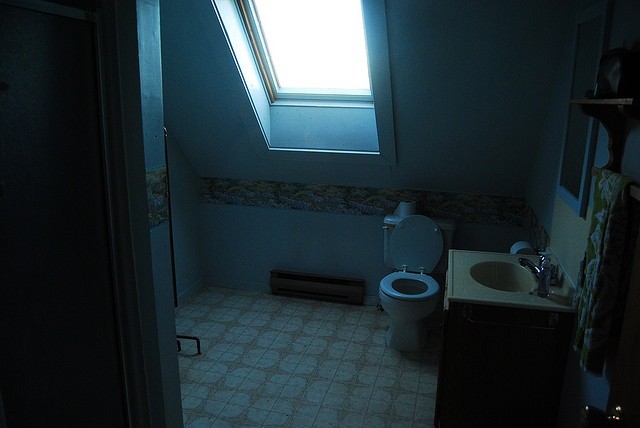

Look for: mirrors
[555,5,609,217]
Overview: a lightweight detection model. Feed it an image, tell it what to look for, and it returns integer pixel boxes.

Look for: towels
[573,168,635,378]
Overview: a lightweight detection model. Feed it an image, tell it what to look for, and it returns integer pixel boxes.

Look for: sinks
[469,260,536,293]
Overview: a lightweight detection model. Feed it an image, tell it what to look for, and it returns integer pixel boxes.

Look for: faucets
[518,252,551,283]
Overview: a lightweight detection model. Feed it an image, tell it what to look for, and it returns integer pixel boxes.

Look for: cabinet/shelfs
[569,97,637,168]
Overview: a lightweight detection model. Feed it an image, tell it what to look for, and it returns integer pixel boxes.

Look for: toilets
[378,214,456,353]
